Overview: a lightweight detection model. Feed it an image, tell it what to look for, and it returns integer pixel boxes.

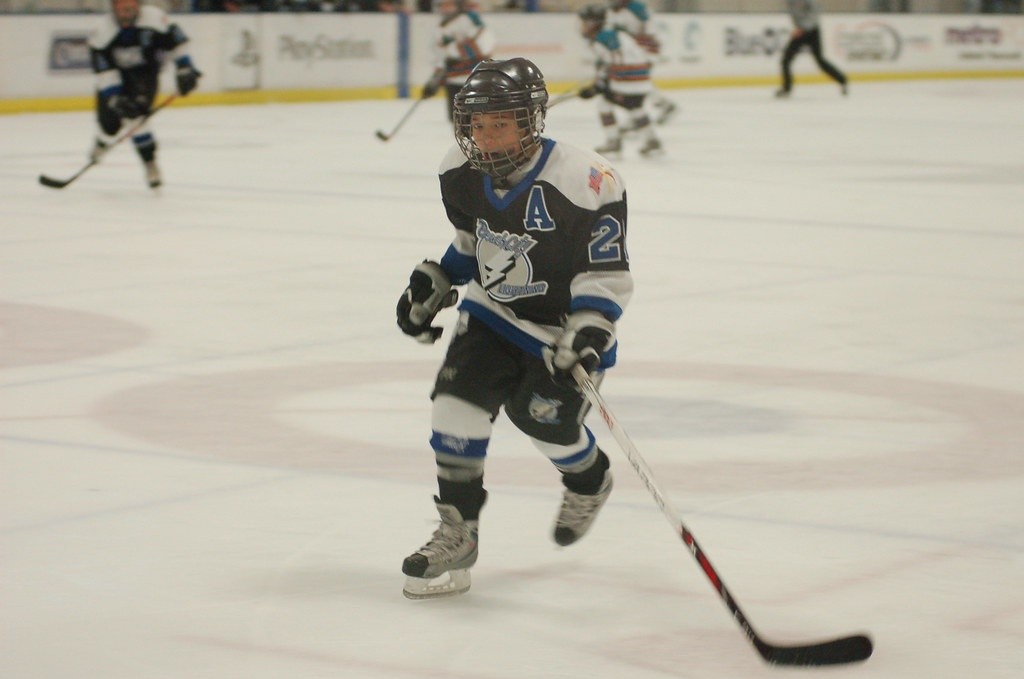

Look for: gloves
[123,95,154,119]
[541,311,615,390]
[178,68,201,95]
[580,84,600,98]
[396,258,458,345]
[421,69,444,99]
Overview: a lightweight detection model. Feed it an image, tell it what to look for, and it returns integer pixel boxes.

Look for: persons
[422,0,497,125]
[775,0,849,98]
[578,0,677,156]
[394,56,634,599]
[92,0,200,188]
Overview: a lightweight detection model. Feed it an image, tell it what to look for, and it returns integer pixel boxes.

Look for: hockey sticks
[37,71,205,188]
[545,87,579,109]
[375,97,424,142]
[568,363,873,667]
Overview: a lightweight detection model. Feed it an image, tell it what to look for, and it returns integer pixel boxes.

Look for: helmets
[453,57,548,139]
[578,4,605,21]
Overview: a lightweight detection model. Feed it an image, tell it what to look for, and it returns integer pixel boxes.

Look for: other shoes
[594,138,621,155]
[146,161,161,187]
[639,138,662,155]
[90,140,107,163]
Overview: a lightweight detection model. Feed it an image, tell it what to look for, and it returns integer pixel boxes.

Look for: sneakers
[402,488,488,600]
[555,469,613,546]
